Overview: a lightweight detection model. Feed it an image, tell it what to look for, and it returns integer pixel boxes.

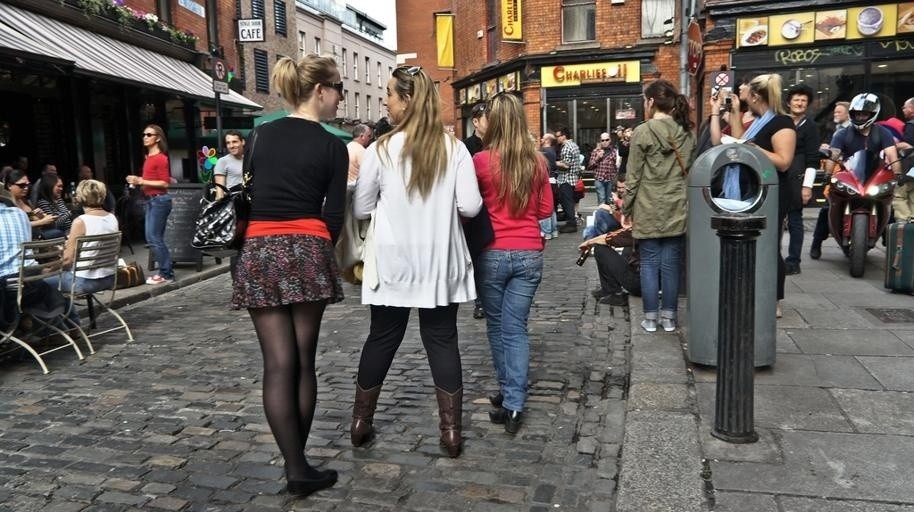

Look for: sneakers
[658,318,676,332]
[811,239,822,259]
[641,318,657,333]
[599,292,628,307]
[146,275,173,285]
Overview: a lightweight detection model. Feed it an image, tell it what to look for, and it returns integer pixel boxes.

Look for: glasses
[15,182,31,189]
[323,80,344,97]
[473,104,486,116]
[407,66,423,78]
[142,133,157,137]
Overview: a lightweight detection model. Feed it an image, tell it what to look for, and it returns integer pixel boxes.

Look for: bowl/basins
[898,6,914,31]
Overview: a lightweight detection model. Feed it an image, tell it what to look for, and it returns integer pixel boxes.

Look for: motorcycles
[819,144,914,279]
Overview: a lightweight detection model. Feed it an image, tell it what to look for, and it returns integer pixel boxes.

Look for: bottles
[575,247,590,267]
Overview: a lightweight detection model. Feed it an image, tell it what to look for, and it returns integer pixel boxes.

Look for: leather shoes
[287,469,337,498]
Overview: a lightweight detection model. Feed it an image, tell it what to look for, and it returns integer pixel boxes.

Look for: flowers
[58,0,202,52]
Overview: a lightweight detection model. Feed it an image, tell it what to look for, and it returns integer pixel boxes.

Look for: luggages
[884,216,914,294]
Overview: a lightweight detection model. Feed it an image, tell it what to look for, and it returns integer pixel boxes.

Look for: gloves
[822,173,832,185]
[897,173,907,187]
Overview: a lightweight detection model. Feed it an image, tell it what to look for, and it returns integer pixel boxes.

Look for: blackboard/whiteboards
[149,183,204,272]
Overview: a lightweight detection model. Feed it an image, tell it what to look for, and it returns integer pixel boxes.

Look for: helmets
[849,92,881,131]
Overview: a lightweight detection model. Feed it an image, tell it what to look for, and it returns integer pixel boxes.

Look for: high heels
[489,393,521,433]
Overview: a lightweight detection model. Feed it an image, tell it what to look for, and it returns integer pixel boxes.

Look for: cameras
[712,91,719,97]
[724,97,732,105]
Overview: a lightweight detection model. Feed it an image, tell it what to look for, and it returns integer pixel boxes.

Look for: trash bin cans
[685,141,779,368]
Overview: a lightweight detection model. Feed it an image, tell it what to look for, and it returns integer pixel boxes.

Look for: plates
[473,85,480,98]
[504,83,515,90]
[780,19,801,40]
[739,24,768,46]
[857,23,881,36]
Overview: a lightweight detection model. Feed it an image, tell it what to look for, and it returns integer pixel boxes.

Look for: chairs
[0,193,136,376]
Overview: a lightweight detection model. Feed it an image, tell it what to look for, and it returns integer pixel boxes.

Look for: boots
[350,381,383,447]
[435,385,463,458]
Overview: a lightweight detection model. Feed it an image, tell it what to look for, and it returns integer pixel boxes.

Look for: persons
[231,50,349,498]
[460,103,491,320]
[811,93,913,280]
[589,124,641,305]
[351,64,483,458]
[2,153,120,355]
[622,82,694,332]
[468,93,555,435]
[528,124,585,234]
[124,124,175,286]
[710,72,821,316]
[211,131,249,285]
[335,116,398,285]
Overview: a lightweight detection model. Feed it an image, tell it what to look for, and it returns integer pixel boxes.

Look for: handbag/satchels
[191,182,248,250]
[116,261,145,289]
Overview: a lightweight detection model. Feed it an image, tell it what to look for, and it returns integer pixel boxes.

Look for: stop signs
[685,20,704,76]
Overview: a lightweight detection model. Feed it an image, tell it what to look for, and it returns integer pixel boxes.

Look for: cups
[597,142,602,150]
[33,208,45,219]
[857,7,883,31]
